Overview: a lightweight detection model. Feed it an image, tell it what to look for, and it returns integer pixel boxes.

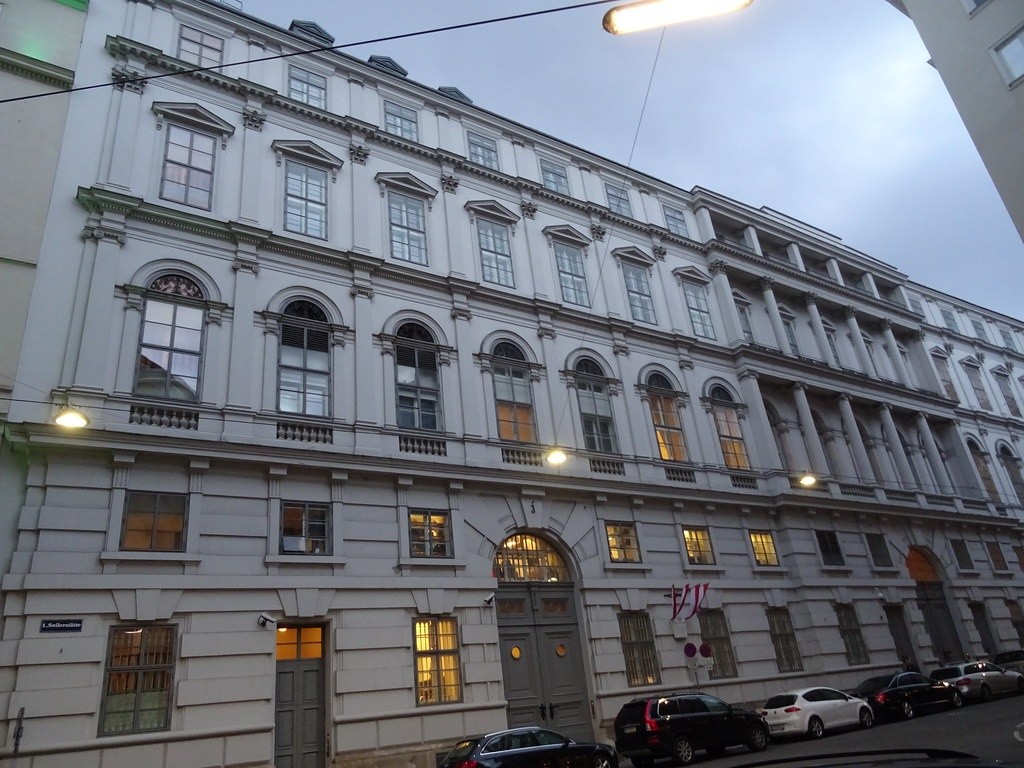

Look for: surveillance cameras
[484,592,495,602]
[261,614,277,623]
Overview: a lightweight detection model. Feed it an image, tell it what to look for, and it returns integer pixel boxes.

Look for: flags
[670,582,710,621]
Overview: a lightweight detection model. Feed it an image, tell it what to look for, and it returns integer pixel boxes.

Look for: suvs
[613,690,769,768]
[437,725,620,768]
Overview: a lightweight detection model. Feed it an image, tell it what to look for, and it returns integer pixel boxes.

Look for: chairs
[519,736,529,748]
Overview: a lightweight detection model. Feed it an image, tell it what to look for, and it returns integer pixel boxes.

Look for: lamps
[545,443,566,464]
[797,472,816,485]
[53,402,91,427]
[602,0,753,36]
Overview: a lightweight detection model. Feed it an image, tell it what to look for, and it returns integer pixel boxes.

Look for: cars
[851,672,963,722]
[759,687,875,739]
[930,661,1024,703]
